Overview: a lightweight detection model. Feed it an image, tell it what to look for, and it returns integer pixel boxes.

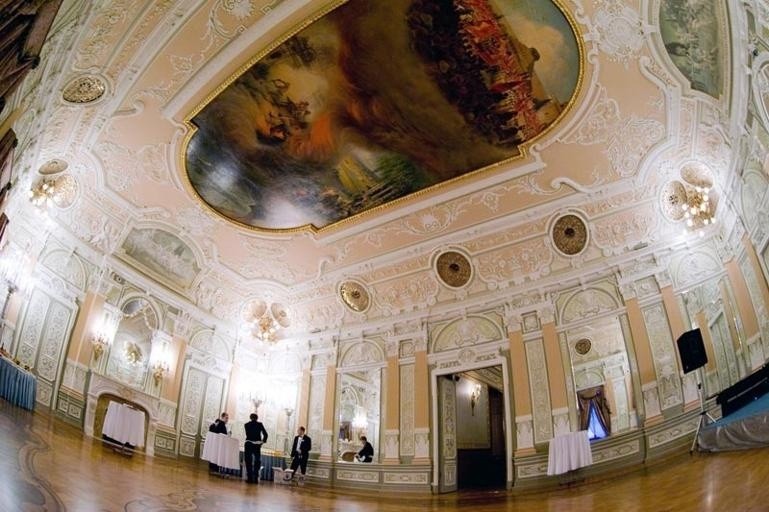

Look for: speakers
[676,328,708,375]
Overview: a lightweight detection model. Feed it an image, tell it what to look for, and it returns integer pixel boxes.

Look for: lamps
[682,183,716,229]
[29,179,60,207]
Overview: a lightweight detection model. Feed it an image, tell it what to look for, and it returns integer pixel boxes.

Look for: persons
[290,426,310,480]
[357,434,373,462]
[242,414,268,484]
[208,412,229,472]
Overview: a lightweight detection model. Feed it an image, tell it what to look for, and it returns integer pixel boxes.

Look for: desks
[201,433,287,481]
[547,430,593,484]
[101,401,145,456]
[0,349,38,412]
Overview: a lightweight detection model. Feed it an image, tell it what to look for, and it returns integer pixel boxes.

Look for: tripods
[689,369,717,456]
